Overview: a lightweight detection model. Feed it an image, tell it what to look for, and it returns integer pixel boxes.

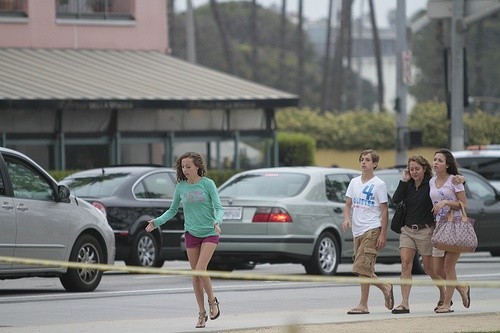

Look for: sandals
[196,311,208,328]
[208,297,220,320]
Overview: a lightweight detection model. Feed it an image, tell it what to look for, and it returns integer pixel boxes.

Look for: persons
[392,149,472,314]
[343,150,395,315]
[145,151,223,328]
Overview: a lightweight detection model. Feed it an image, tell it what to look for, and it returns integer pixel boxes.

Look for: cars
[0,148,118,292]
[58,163,189,270]
[451,144,500,194]
[378,167,500,258]
[207,165,426,276]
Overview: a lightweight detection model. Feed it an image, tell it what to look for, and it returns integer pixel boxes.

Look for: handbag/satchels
[391,201,406,233]
[431,201,478,253]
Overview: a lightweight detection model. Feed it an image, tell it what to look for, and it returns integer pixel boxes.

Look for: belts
[405,223,435,228]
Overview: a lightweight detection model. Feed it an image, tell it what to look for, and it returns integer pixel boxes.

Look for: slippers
[392,305,409,313]
[434,301,453,311]
[385,284,394,310]
[436,308,454,313]
[463,286,470,308]
[347,308,369,314]
